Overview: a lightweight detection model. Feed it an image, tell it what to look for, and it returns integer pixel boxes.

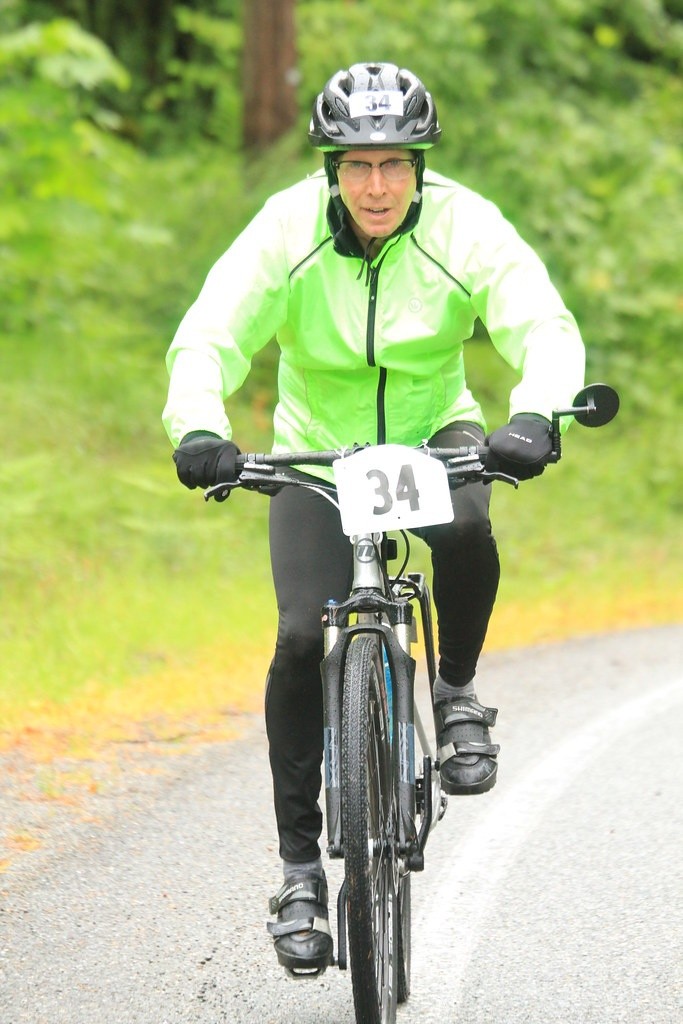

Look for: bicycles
[204,383,620,1024]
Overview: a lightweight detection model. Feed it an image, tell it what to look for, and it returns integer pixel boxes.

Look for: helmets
[307,63,442,152]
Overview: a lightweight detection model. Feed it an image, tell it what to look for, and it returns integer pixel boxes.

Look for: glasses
[332,156,419,181]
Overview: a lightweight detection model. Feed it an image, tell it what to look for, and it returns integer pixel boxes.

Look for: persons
[161,62,586,967]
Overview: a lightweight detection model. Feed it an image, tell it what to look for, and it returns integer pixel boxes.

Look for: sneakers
[432,694,500,796]
[267,867,334,968]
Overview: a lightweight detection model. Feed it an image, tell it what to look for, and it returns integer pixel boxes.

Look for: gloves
[171,429,243,502]
[481,412,554,485]
